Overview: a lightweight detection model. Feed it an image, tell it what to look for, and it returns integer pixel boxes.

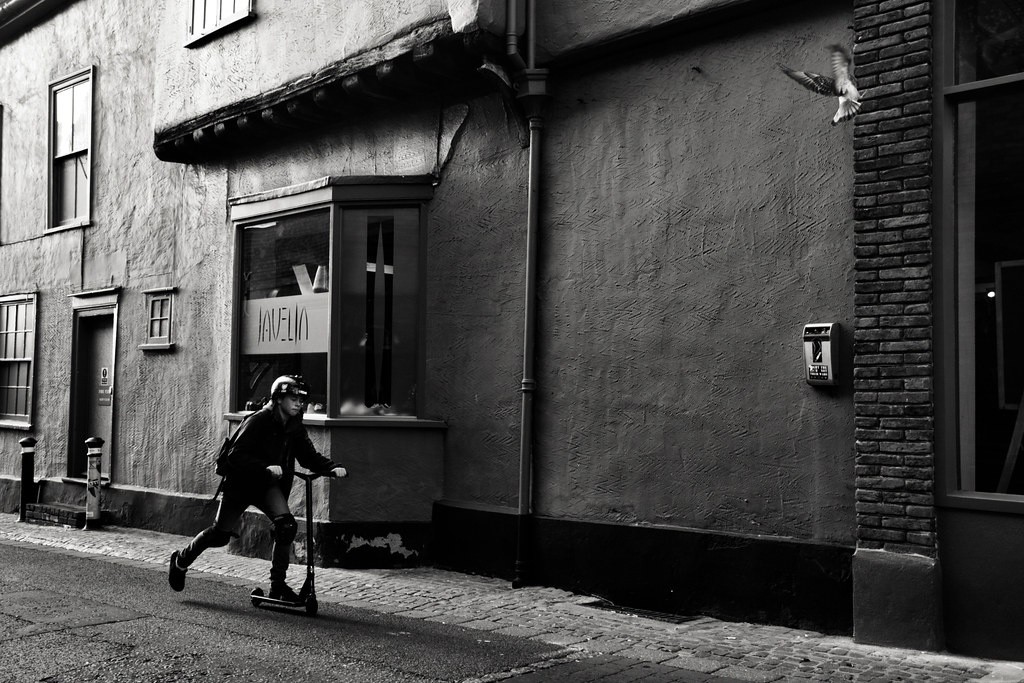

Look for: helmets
[270,375,311,403]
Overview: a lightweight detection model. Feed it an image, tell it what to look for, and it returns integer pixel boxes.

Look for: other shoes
[168,550,186,591]
[268,582,299,603]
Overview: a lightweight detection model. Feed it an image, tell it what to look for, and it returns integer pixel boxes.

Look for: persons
[169,373,347,605]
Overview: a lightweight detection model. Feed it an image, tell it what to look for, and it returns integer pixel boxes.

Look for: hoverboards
[248,468,351,617]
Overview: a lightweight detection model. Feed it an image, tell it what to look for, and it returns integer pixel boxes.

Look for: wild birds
[775,44,868,127]
